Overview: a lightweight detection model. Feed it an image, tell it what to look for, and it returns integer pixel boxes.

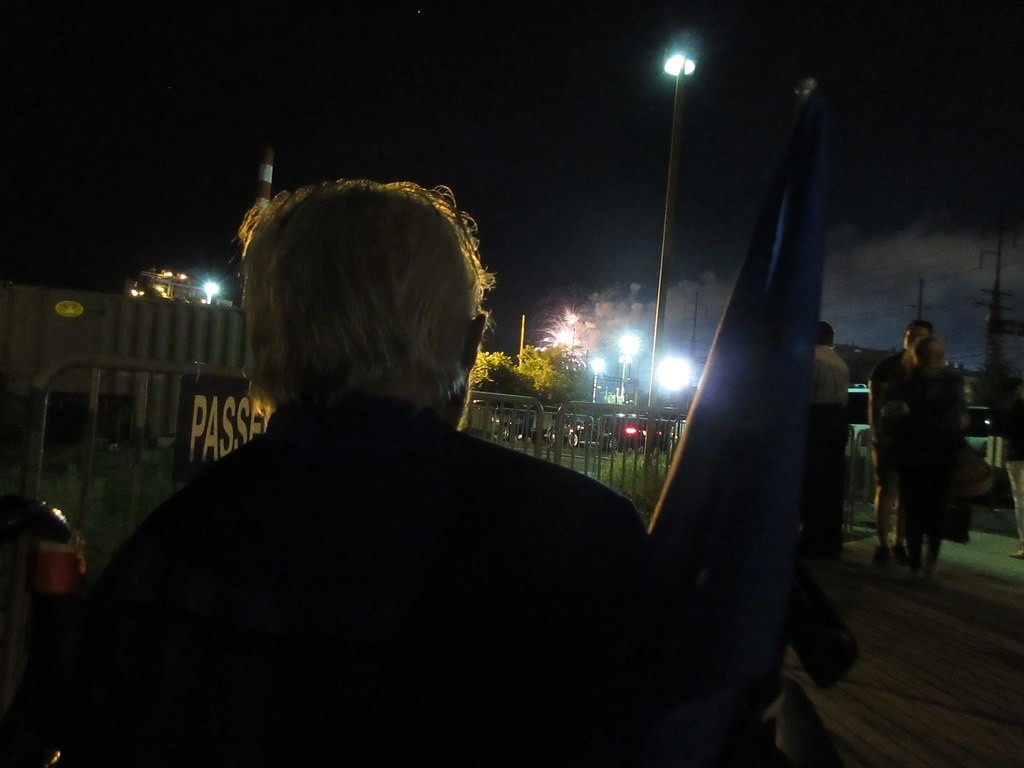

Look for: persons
[801,319,849,558]
[45,179,695,768]
[1000,378,1024,559]
[866,318,973,591]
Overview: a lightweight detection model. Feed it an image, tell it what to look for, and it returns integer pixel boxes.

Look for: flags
[650,90,827,733]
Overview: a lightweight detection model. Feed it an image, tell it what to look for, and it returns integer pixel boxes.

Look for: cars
[963,405,1009,464]
[846,387,872,460]
[473,400,670,452]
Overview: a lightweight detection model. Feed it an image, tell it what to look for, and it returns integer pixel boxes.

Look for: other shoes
[894,543,910,563]
[875,545,889,565]
[897,572,920,587]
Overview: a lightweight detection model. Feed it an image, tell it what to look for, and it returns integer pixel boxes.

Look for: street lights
[640,28,702,501]
[591,356,605,404]
[616,332,640,401]
